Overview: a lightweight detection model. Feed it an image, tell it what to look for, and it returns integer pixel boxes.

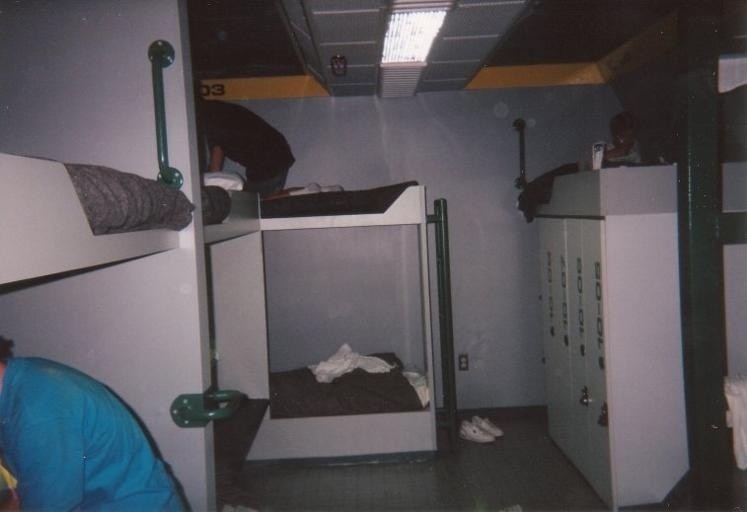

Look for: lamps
[379,0,457,67]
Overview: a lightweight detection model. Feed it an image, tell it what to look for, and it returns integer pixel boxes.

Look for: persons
[0,336,192,511]
[197,94,342,201]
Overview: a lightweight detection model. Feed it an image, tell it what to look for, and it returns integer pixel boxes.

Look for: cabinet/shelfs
[535,162,747,512]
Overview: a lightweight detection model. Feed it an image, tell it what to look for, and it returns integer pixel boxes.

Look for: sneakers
[459,416,504,443]
